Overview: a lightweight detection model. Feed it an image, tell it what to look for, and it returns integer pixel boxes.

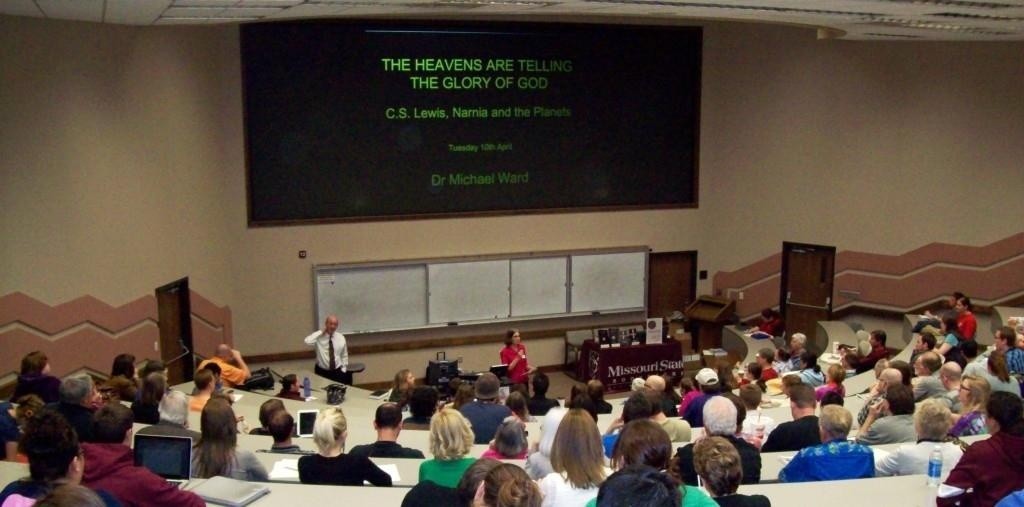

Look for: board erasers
[447,322,458,326]
[592,311,600,315]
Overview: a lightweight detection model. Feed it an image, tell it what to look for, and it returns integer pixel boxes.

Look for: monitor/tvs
[490,364,508,379]
[637,330,646,343]
[663,327,668,339]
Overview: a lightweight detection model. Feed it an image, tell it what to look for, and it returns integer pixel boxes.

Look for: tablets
[297,409,319,438]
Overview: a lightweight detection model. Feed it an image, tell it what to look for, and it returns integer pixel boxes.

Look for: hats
[474,371,500,401]
[630,376,647,391]
[692,365,721,388]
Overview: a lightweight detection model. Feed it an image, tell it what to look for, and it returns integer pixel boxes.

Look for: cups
[751,422,765,439]
[832,341,839,353]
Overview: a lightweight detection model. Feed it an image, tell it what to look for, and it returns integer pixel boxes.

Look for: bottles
[927,443,942,488]
[301,375,311,396]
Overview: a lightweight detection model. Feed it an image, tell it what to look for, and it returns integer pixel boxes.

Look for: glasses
[75,444,89,459]
[958,382,972,392]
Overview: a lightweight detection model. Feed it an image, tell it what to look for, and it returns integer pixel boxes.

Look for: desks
[0,303,1023,506]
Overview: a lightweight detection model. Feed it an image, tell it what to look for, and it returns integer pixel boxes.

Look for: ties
[326,335,336,370]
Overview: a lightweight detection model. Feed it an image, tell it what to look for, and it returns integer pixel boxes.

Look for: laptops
[187,475,269,506]
[133,434,192,490]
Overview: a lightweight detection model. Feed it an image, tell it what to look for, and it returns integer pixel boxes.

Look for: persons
[198,344,251,389]
[249,398,315,455]
[276,374,305,401]
[0,291,1024,507]
[1,351,269,506]
[388,368,773,506]
[298,406,393,487]
[756,292,1022,506]
[349,402,424,458]
[499,328,530,399]
[304,316,348,384]
